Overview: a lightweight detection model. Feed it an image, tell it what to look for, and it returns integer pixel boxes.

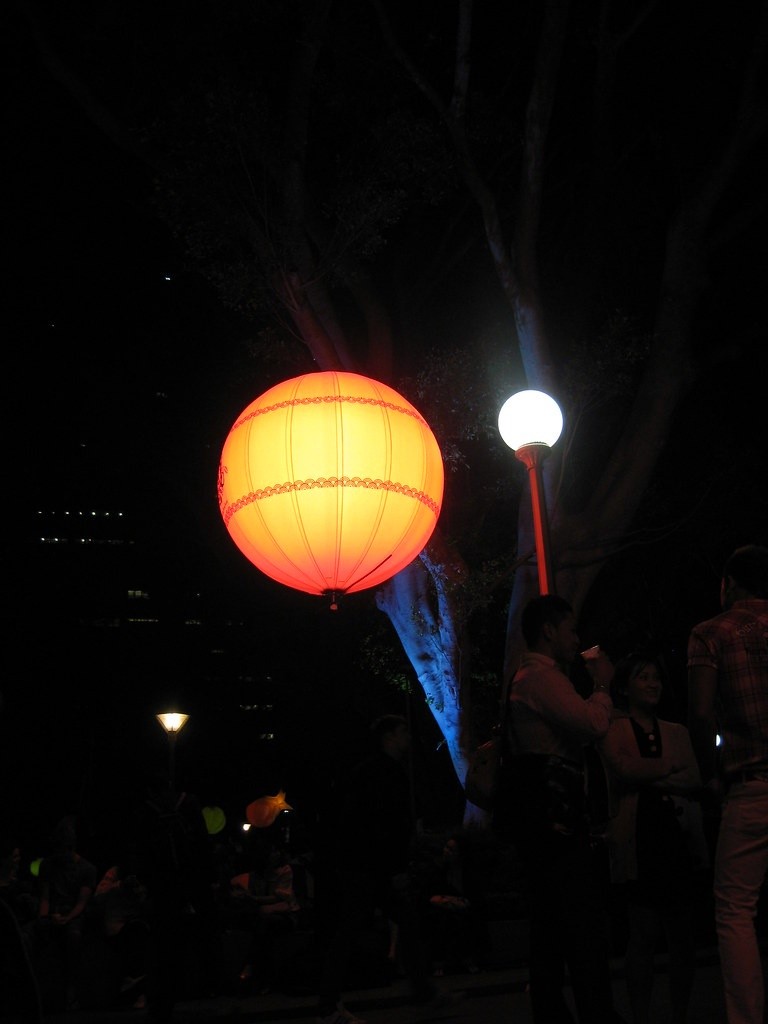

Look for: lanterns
[216,371,445,610]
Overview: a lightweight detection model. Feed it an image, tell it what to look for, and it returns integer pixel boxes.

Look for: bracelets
[594,681,609,691]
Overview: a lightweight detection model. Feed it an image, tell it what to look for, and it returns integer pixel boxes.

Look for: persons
[502,594,631,1024]
[599,653,713,1024]
[686,543,768,1024]
[0,714,501,1024]
